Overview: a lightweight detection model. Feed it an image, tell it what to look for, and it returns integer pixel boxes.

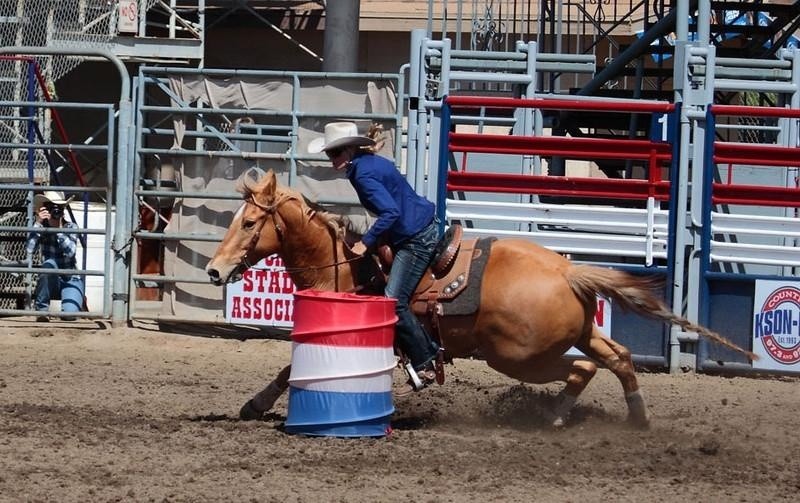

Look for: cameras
[42,201,64,219]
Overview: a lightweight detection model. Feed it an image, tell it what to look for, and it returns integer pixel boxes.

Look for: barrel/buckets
[284,289,398,437]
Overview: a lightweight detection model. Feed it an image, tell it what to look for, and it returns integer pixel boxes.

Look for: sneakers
[396,362,437,397]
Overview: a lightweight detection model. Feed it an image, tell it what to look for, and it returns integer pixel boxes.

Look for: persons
[307,120,445,398]
[25,190,85,323]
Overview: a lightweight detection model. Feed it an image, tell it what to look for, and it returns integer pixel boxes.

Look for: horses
[204,169,764,433]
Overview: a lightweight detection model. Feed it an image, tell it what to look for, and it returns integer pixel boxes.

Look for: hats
[307,122,377,154]
[34,190,75,208]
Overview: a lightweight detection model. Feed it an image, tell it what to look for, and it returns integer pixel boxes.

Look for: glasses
[325,147,345,158]
